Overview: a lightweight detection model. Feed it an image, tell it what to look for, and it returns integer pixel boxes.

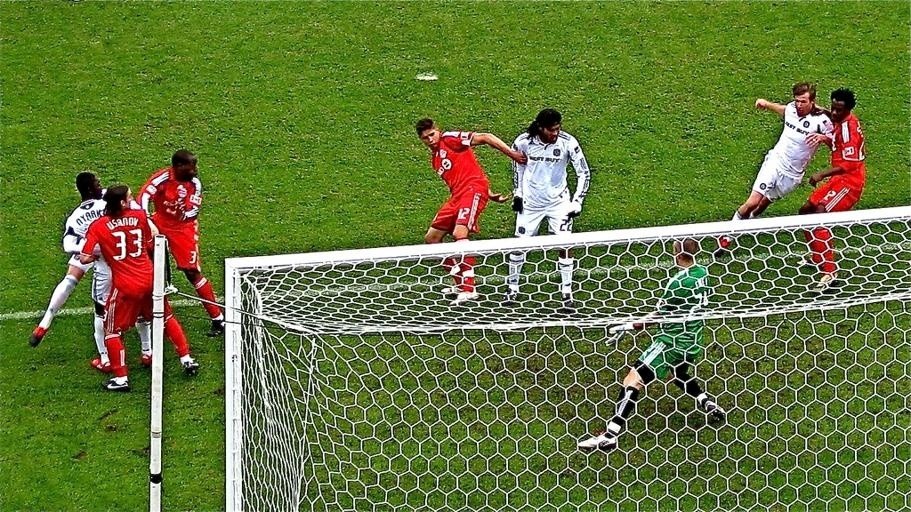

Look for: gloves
[565,201,583,218]
[175,197,186,222]
[511,196,524,214]
[605,330,627,348]
[609,324,637,336]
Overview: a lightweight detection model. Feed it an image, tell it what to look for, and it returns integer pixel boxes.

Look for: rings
[813,138,817,143]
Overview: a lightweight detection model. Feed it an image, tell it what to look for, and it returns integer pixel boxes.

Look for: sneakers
[704,400,728,424]
[91,349,201,392]
[207,317,226,338]
[578,434,620,450]
[713,237,731,259]
[30,327,50,348]
[449,291,479,306]
[441,285,464,295]
[797,258,838,293]
[556,289,575,317]
[503,286,520,306]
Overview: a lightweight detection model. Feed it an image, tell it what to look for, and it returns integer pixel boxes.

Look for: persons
[798,87,866,293]
[62,172,153,374]
[27,188,161,349]
[417,118,527,304]
[78,183,199,392]
[720,81,835,248]
[503,109,592,308]
[134,148,226,339]
[578,238,728,450]
